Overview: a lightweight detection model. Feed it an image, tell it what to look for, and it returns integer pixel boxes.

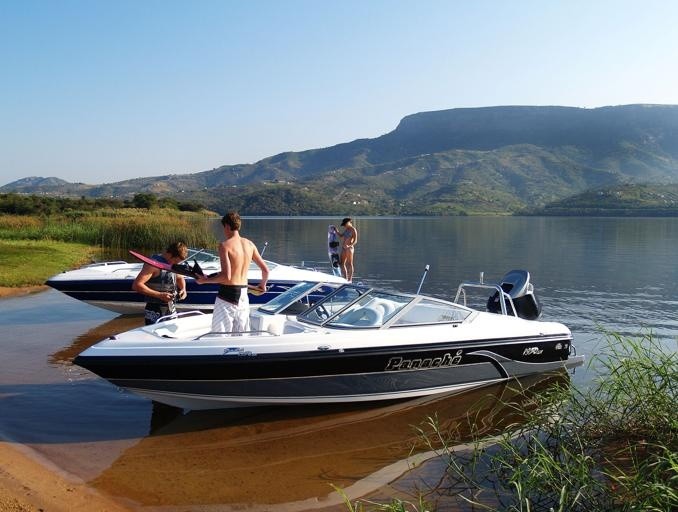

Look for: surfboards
[327,225,342,277]
[130,250,263,293]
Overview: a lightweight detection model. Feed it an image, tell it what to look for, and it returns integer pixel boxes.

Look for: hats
[340,217,352,227]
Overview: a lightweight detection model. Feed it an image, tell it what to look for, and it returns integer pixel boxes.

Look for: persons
[132,241,189,325]
[195,210,270,337]
[331,218,358,283]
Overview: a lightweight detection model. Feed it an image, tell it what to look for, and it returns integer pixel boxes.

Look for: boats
[43,242,353,316]
[72,263,587,415]
